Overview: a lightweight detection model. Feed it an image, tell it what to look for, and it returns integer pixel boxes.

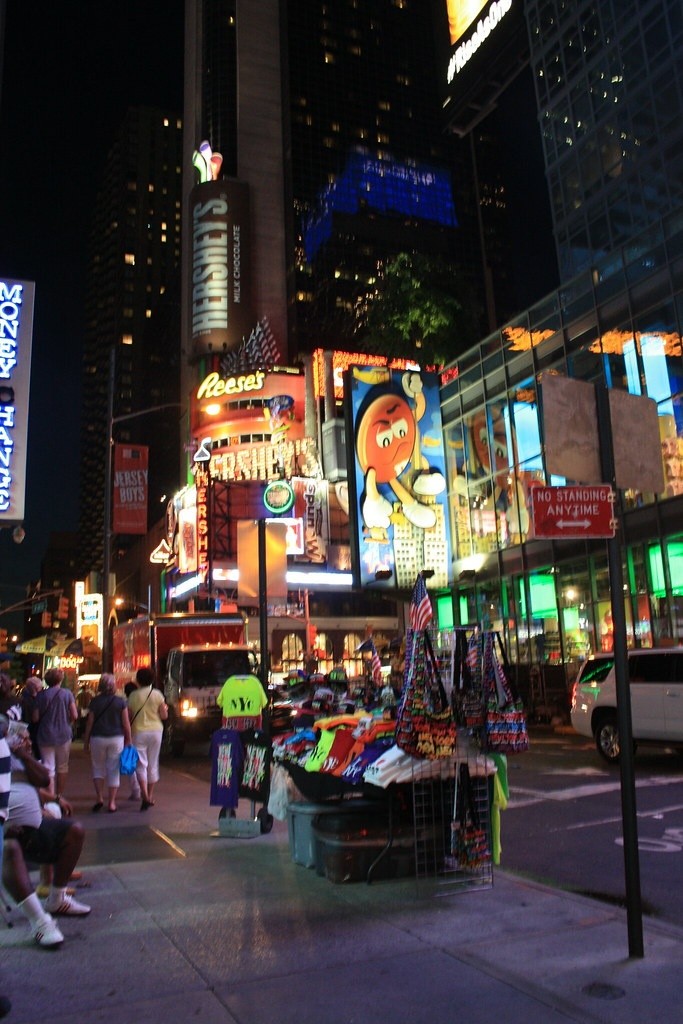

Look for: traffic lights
[58,597,70,621]
[41,610,53,628]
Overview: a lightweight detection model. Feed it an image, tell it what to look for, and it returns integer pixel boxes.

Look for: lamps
[365,570,392,587]
[421,569,434,581]
[448,569,476,588]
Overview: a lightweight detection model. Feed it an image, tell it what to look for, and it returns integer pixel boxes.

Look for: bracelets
[56,794,61,803]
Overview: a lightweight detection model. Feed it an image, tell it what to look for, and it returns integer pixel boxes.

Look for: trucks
[110,610,264,758]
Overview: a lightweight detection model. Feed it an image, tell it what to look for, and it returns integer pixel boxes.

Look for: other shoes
[36,885,77,897]
[138,799,154,812]
[129,795,140,802]
[68,872,82,881]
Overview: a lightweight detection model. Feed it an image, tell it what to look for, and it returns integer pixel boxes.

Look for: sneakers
[31,910,65,947]
[42,895,92,916]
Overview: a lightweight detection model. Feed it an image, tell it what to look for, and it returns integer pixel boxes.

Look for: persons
[83,677,132,812]
[37,668,78,796]
[74,686,93,740]
[125,669,169,812]
[0,674,91,946]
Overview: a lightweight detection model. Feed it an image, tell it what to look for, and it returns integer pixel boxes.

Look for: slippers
[109,805,118,812]
[92,801,103,813]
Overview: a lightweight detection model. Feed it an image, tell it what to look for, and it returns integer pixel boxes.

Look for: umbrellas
[14,634,63,689]
[50,637,103,669]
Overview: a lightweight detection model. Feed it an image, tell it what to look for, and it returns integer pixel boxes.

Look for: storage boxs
[284,799,381,869]
[308,814,434,885]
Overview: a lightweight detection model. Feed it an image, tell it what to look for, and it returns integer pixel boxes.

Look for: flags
[369,640,383,686]
[262,316,282,363]
[407,576,433,632]
[466,636,478,668]
[219,341,247,377]
[255,327,273,365]
[246,335,262,369]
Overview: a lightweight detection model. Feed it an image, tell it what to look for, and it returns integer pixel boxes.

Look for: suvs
[570,646,683,764]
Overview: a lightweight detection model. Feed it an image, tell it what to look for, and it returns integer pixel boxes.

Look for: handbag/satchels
[119,743,138,776]
[396,630,530,759]
